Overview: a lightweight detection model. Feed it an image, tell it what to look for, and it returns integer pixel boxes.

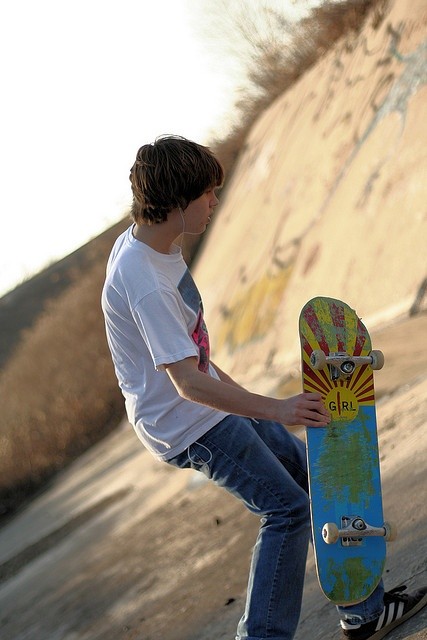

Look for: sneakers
[339,587,427,640]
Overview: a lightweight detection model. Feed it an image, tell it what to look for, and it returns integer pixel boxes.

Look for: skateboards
[299,297,398,606]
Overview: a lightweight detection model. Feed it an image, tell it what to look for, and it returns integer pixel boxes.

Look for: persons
[101,139,427,640]
